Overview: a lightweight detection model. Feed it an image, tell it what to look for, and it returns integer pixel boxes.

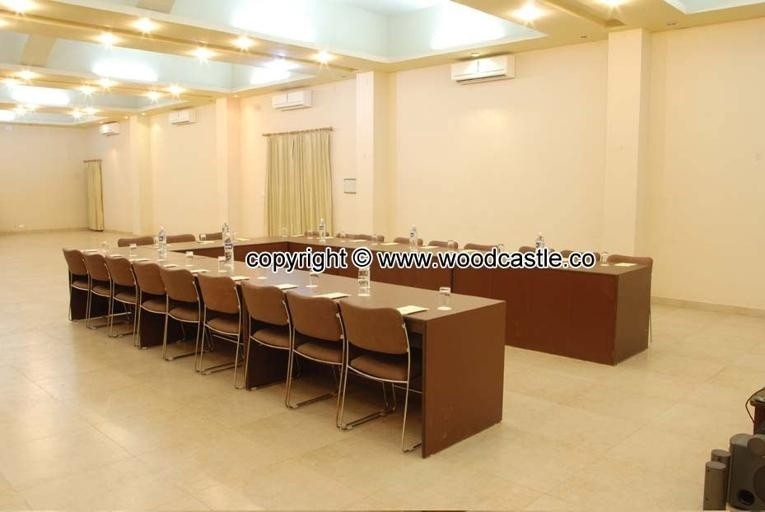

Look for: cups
[309,270,319,286]
[339,228,347,242]
[600,250,609,263]
[306,226,314,239]
[371,233,378,246]
[438,287,453,310]
[448,240,456,253]
[130,233,225,272]
[282,224,289,237]
[496,240,504,253]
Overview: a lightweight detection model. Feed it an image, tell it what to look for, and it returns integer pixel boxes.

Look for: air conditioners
[168,107,197,125]
[270,88,314,111]
[453,53,515,84]
[100,123,121,135]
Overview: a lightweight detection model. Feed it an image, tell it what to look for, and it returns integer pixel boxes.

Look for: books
[151,237,251,246]
[76,247,249,282]
[396,305,428,316]
[290,230,639,269]
[312,291,350,301]
[275,283,297,290]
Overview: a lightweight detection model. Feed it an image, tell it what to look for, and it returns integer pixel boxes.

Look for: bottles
[408,225,418,253]
[225,236,235,266]
[158,226,168,261]
[317,219,327,242]
[356,259,371,297]
[221,222,231,240]
[536,233,544,252]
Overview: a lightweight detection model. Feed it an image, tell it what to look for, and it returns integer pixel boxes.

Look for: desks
[126,235,289,259]
[452,253,650,366]
[287,237,452,288]
[73,248,506,456]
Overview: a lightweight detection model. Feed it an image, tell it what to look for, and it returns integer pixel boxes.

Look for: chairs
[132,262,173,355]
[340,302,422,456]
[62,248,138,339]
[118,232,230,247]
[239,282,301,407]
[287,290,342,424]
[197,272,242,390]
[160,265,204,374]
